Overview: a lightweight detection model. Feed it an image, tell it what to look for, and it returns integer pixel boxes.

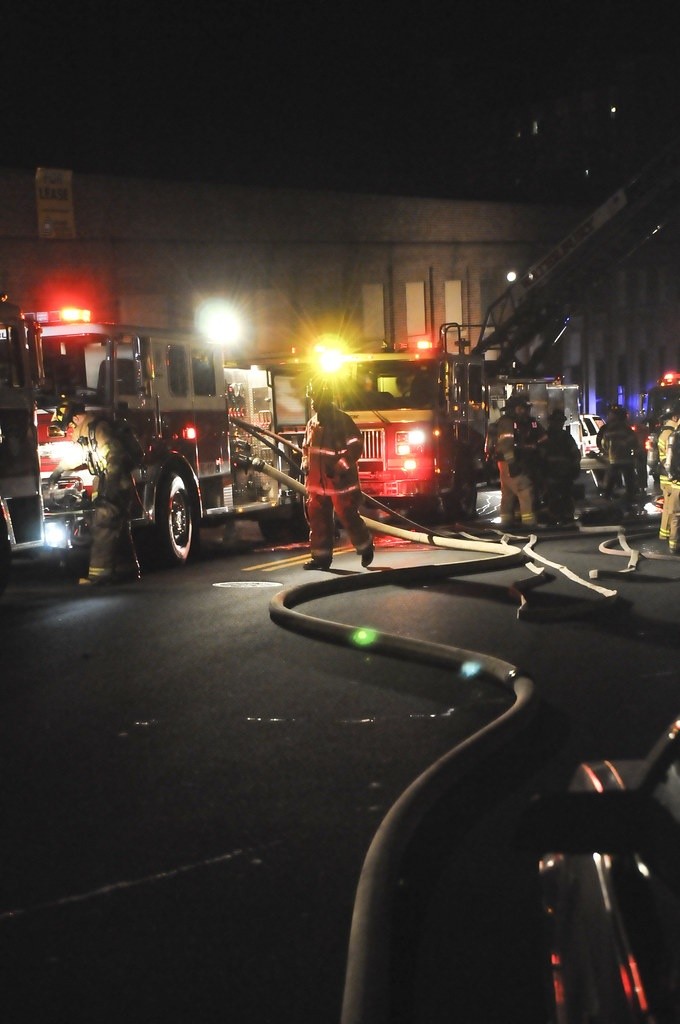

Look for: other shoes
[304,558,332,570]
[599,490,613,499]
[669,548,680,555]
[79,575,106,587]
[360,541,373,567]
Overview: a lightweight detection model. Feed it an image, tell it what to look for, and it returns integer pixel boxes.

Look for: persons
[496,395,581,533]
[596,405,641,500]
[658,406,680,554]
[47,399,145,584]
[300,376,374,571]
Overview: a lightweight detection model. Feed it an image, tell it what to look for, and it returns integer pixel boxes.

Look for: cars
[573,413,607,457]
[510,711,679,1024]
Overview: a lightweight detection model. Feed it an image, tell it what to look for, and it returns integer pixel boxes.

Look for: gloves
[508,463,521,477]
[300,457,309,476]
[102,480,117,501]
[48,470,61,486]
[335,458,350,474]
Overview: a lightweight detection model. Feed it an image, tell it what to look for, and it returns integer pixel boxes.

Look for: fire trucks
[0,146,680,585]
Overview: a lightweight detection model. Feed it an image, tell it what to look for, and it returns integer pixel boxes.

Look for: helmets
[548,410,567,422]
[499,396,531,411]
[658,399,680,423]
[610,403,625,414]
[306,379,331,396]
[56,399,85,430]
